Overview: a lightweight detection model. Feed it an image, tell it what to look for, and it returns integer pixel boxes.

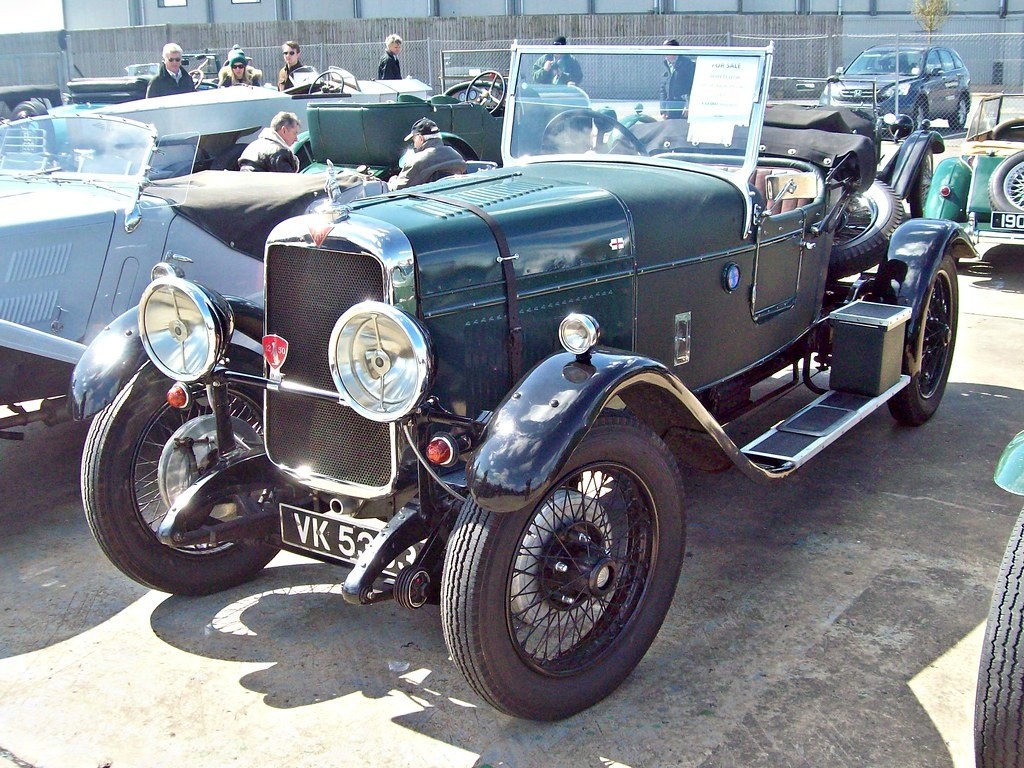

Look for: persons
[277,40,305,93]
[217,43,263,87]
[387,117,468,192]
[532,35,583,87]
[377,34,403,79]
[659,38,695,119]
[237,111,303,175]
[145,42,197,98]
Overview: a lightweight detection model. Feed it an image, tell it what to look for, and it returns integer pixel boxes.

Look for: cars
[61,42,986,722]
[969,429,1024,768]
[918,86,1024,275]
[0,50,679,450]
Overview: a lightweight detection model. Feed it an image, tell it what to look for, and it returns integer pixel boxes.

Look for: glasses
[167,56,181,62]
[283,51,296,55]
[232,65,246,69]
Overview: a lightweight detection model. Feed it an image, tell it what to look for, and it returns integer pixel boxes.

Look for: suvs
[819,41,973,137]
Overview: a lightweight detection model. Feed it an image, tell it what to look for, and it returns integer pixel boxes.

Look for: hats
[404,118,440,140]
[664,38,680,46]
[228,50,247,72]
[233,44,245,57]
[551,36,566,45]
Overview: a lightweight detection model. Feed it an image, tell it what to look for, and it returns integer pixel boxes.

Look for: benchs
[651,152,813,215]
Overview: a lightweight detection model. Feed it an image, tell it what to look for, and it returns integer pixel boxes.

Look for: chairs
[397,93,461,104]
